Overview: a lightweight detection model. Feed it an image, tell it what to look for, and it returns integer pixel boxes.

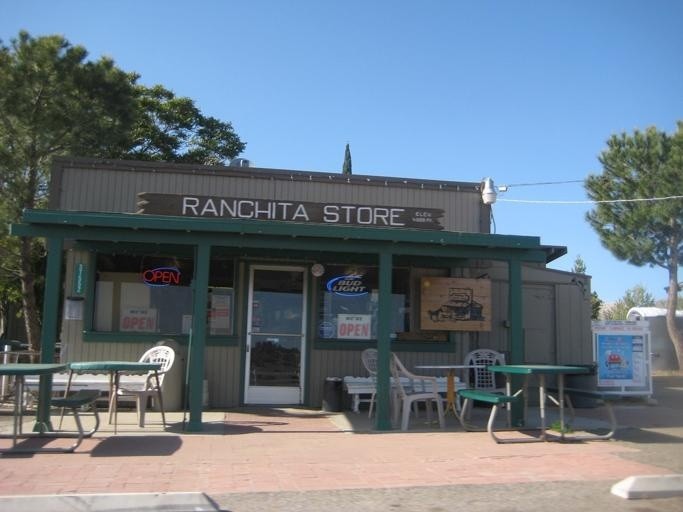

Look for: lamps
[481,179,497,206]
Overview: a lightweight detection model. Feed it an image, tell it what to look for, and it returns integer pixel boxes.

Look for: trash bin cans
[567,363,599,408]
[322,377,342,411]
[151,338,184,412]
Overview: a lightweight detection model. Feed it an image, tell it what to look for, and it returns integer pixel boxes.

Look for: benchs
[546,386,641,440]
[344,375,467,413]
[458,391,518,443]
[14,373,154,412]
[50,389,102,453]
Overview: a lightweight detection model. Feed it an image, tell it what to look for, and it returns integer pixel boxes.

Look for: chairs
[465,349,506,408]
[108,345,175,428]
[362,348,446,431]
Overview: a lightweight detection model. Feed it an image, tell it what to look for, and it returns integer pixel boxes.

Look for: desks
[1,363,66,453]
[415,365,486,429]
[57,361,168,434]
[487,364,590,443]
